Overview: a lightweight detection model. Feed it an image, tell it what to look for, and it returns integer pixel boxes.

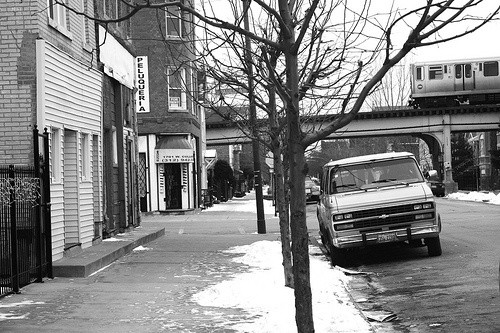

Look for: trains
[407,57,500,109]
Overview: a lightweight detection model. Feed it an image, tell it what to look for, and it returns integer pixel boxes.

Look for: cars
[305,176,321,204]
[426,176,446,197]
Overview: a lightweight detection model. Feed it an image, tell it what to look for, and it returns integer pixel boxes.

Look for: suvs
[317,152,443,266]
